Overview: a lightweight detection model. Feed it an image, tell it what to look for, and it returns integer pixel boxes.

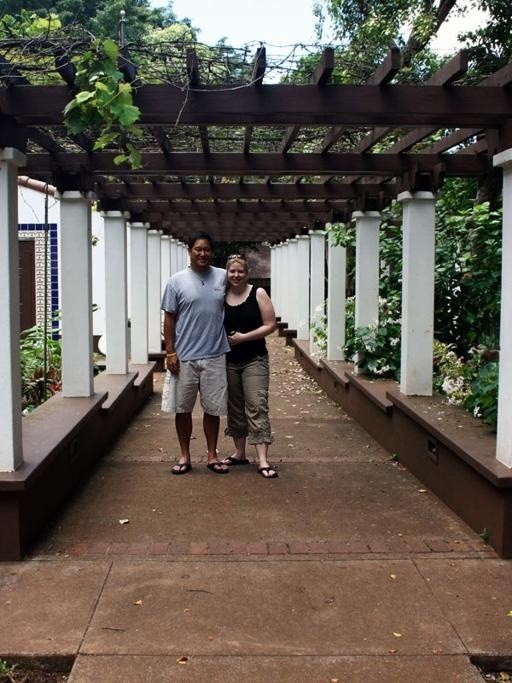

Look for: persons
[222,254,277,478]
[161,232,232,474]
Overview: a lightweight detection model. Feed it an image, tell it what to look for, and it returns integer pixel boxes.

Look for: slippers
[171,463,192,474]
[257,466,278,478]
[207,462,229,473]
[221,456,249,466]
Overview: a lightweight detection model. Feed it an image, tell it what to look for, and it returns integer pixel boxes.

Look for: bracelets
[166,353,177,357]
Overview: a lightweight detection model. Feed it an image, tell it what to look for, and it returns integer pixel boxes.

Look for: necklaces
[199,279,206,286]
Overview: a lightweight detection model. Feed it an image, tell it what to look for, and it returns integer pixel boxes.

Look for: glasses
[227,254,247,260]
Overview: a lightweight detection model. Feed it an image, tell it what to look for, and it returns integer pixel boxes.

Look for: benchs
[273,315,512,557]
[0,334,168,561]
